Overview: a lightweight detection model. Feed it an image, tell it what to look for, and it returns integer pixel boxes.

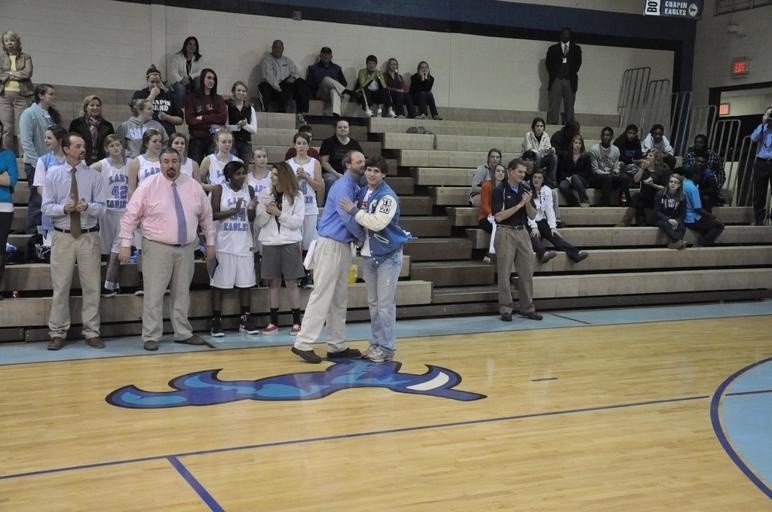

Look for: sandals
[573,253,588,262]
[540,251,557,263]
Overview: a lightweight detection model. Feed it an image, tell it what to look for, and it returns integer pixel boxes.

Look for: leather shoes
[47,337,64,349]
[501,313,512,321]
[290,346,322,364]
[85,336,106,349]
[144,341,158,351]
[173,335,207,345]
[327,347,361,359]
[525,312,543,320]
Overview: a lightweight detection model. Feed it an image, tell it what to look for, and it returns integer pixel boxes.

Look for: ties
[170,183,188,248]
[69,167,81,240]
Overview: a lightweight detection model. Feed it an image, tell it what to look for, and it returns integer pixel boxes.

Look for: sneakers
[301,273,314,289]
[239,307,260,335]
[135,271,144,296]
[362,344,378,358]
[368,346,397,362]
[100,288,117,298]
[289,324,301,336]
[263,323,280,334]
[211,310,224,336]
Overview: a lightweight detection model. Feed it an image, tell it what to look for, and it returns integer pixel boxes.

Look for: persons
[589,127,622,206]
[116,100,167,160]
[652,175,687,246]
[615,123,645,186]
[751,108,771,225]
[684,168,724,246]
[258,162,304,336]
[633,148,671,224]
[125,129,168,203]
[640,125,676,179]
[222,82,256,163]
[0,31,36,153]
[171,35,202,100]
[305,46,357,120]
[470,148,500,210]
[36,126,86,260]
[320,121,365,198]
[545,27,582,125]
[407,61,440,119]
[262,40,310,129]
[291,153,365,363]
[685,135,727,194]
[491,160,543,321]
[184,70,226,160]
[284,133,324,288]
[208,160,259,336]
[518,152,536,181]
[128,63,184,144]
[0,121,18,296]
[340,158,417,362]
[247,150,274,195]
[688,153,724,215]
[477,165,506,262]
[359,55,397,117]
[21,85,61,264]
[522,117,559,187]
[560,136,591,207]
[69,97,114,163]
[115,152,214,351]
[383,58,412,116]
[88,135,134,299]
[198,130,244,198]
[42,134,105,350]
[527,173,589,264]
